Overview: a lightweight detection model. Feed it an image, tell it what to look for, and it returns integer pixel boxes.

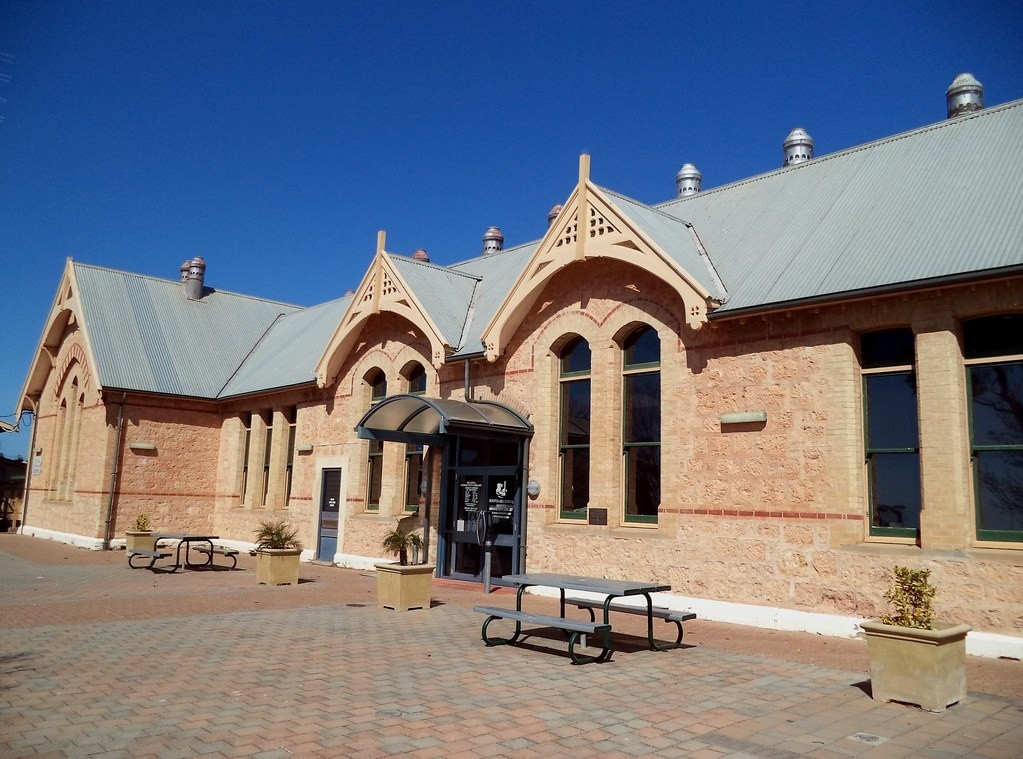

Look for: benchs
[127,548,173,575]
[472,606,613,665]
[564,596,697,651]
[192,547,239,571]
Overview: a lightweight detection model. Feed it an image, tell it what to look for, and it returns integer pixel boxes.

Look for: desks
[502,573,671,664]
[149,532,220,575]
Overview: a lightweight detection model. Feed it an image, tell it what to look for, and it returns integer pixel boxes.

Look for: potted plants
[374,518,437,611]
[254,520,304,587]
[124,514,156,557]
[860,564,974,713]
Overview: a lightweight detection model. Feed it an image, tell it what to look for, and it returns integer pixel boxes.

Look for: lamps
[721,411,767,424]
[296,444,313,451]
[129,442,156,449]
